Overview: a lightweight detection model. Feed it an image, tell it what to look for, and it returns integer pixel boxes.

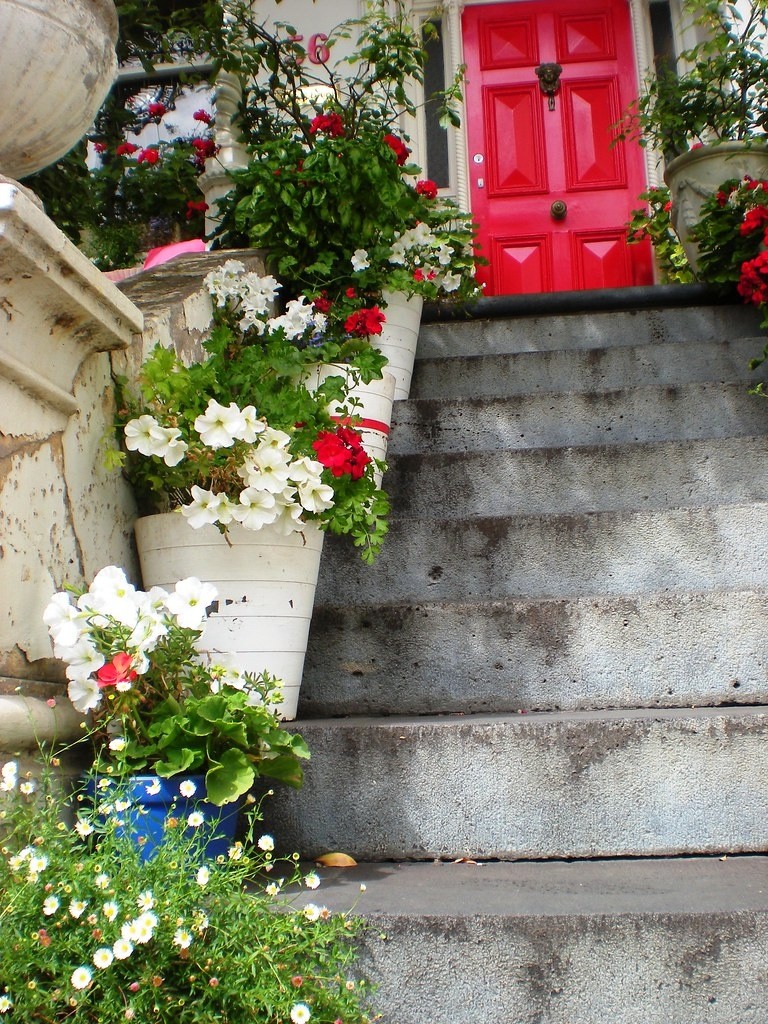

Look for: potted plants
[603,0,768,279]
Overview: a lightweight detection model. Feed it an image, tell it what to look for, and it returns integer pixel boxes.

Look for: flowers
[100,257,388,568]
[33,108,164,262]
[0,562,387,1024]
[145,0,485,351]
[685,172,768,317]
